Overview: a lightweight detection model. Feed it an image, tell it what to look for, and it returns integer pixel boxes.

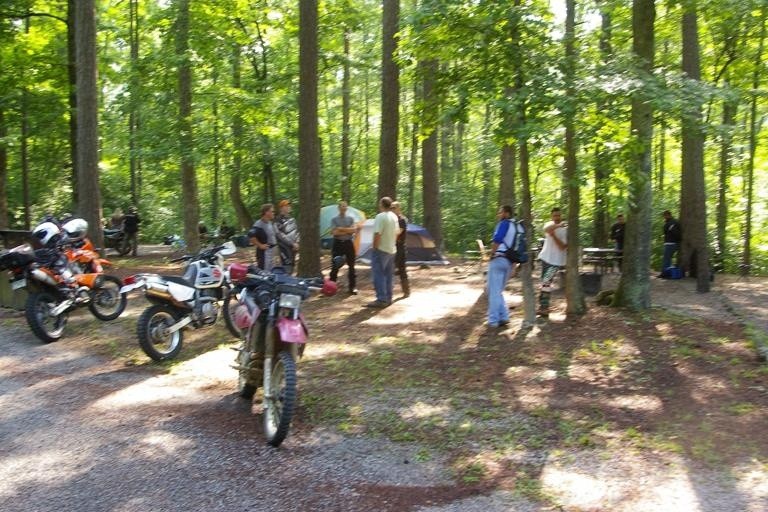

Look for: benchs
[577,255,625,276]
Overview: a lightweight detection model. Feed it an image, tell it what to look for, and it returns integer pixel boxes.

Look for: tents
[314,204,370,248]
[352,219,452,267]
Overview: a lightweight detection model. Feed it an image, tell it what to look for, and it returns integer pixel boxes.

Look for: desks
[531,246,624,276]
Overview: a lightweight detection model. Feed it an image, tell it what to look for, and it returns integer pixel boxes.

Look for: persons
[119,204,141,256]
[535,208,570,324]
[328,199,361,297]
[660,208,682,272]
[110,208,125,231]
[247,203,283,274]
[272,199,301,276]
[607,213,627,268]
[197,221,209,234]
[368,196,401,310]
[219,221,230,234]
[389,200,411,300]
[483,203,527,330]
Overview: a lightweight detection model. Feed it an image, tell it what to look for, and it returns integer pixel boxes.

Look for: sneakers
[535,307,550,317]
[350,288,358,294]
[368,300,389,308]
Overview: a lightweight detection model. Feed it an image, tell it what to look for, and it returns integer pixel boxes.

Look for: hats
[279,200,292,207]
[32,221,60,246]
[62,219,89,241]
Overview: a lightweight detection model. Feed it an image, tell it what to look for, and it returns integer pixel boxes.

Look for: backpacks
[498,219,529,263]
[662,266,684,279]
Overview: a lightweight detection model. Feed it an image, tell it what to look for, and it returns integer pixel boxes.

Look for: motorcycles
[7,223,146,345]
[121,248,261,362]
[221,264,343,455]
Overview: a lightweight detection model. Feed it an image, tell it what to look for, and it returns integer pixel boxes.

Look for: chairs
[475,239,492,273]
